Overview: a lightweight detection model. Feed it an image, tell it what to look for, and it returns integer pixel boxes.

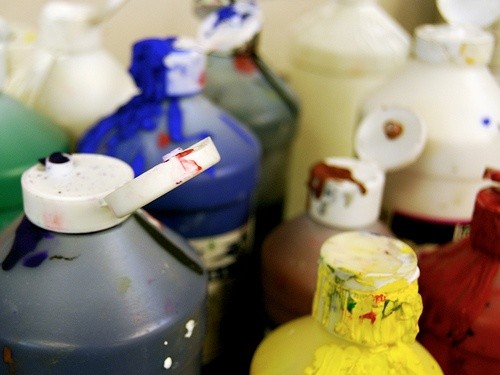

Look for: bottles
[1,1,498,373]
